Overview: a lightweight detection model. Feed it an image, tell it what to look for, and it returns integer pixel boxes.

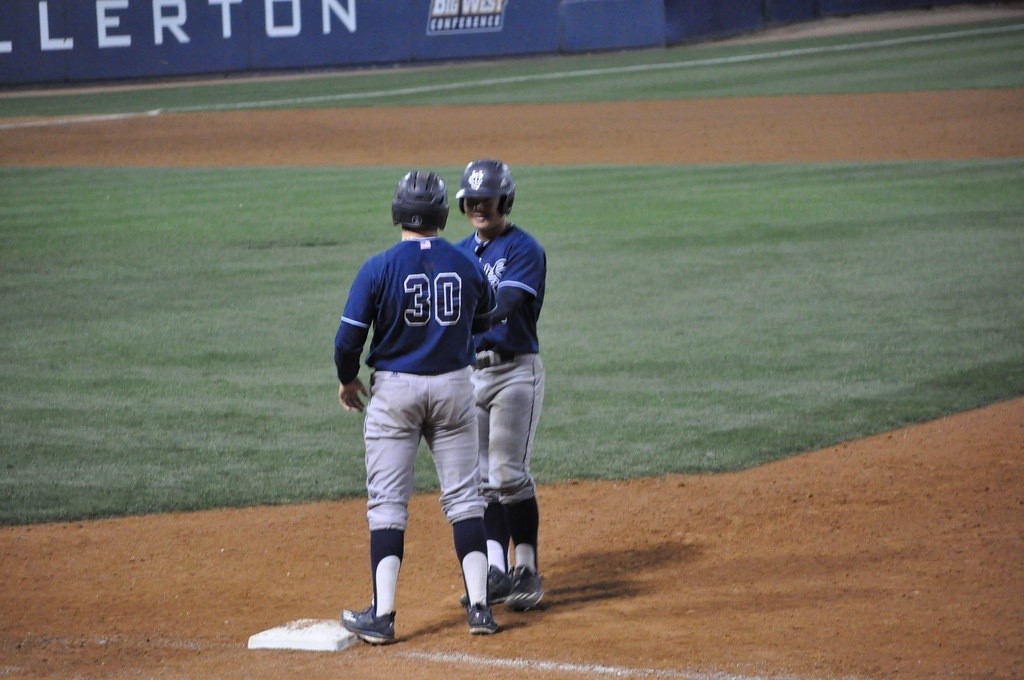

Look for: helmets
[456,160,515,216]
[390,169,450,230]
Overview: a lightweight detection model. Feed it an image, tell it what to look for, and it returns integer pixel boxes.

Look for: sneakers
[460,565,511,606]
[503,565,544,610]
[340,603,397,645]
[465,603,499,633]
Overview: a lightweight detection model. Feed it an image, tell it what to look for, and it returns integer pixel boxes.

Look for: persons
[453,160,547,609]
[333,171,498,642]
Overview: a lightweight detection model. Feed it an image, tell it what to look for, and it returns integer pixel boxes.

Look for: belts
[472,351,515,370]
